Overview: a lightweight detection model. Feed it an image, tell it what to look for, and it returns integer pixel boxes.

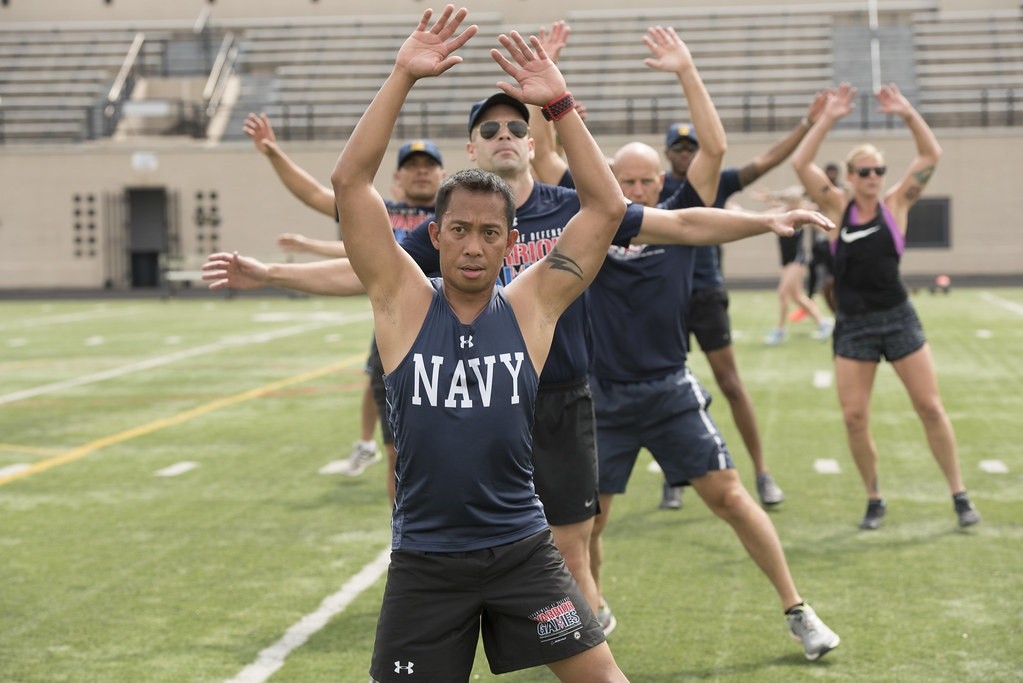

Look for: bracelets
[538,91,577,123]
[801,117,815,129]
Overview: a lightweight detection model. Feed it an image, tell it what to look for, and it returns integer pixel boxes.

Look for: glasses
[670,142,697,153]
[855,167,886,177]
[474,120,531,140]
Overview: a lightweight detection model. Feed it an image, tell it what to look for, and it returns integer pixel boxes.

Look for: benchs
[0,5,1023,143]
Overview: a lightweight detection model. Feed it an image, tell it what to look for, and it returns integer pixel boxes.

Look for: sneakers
[660,480,683,507]
[953,491,979,526]
[755,470,783,504]
[596,597,617,635]
[344,443,381,477]
[787,604,840,660]
[858,498,887,529]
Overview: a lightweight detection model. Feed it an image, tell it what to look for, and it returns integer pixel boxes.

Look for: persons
[331,4,631,683]
[793,82,983,532]
[522,21,842,663]
[200,97,837,621]
[244,91,842,636]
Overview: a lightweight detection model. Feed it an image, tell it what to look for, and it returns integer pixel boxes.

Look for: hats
[397,140,442,171]
[467,93,530,139]
[666,122,699,145]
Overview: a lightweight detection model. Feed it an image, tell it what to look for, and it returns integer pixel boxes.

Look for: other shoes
[764,327,787,344]
[814,317,833,339]
